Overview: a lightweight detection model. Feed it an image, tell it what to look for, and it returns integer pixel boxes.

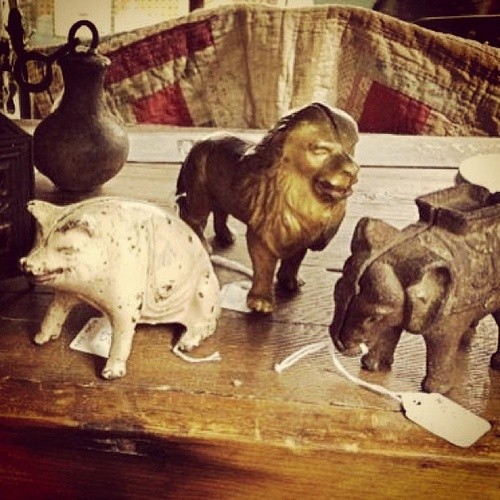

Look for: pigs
[18,197,223,379]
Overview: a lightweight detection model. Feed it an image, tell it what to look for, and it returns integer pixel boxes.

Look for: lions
[174,100,359,315]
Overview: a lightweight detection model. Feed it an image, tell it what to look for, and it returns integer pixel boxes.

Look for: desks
[0,118,499,499]
